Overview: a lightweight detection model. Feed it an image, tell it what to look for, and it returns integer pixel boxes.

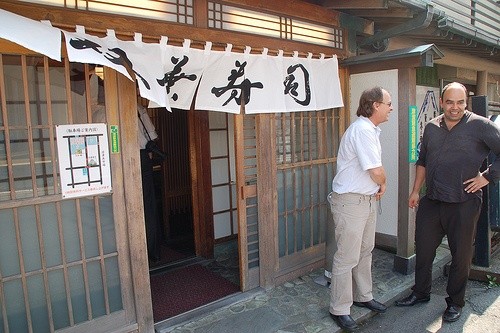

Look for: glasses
[378,101,392,107]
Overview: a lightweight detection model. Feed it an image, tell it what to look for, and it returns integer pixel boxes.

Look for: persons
[395,81,500,322]
[327,86,394,330]
[136,103,162,264]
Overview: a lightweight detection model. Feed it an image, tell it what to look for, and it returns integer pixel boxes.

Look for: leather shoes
[353,299,386,312]
[395,291,430,306]
[442,301,462,322]
[330,313,359,331]
